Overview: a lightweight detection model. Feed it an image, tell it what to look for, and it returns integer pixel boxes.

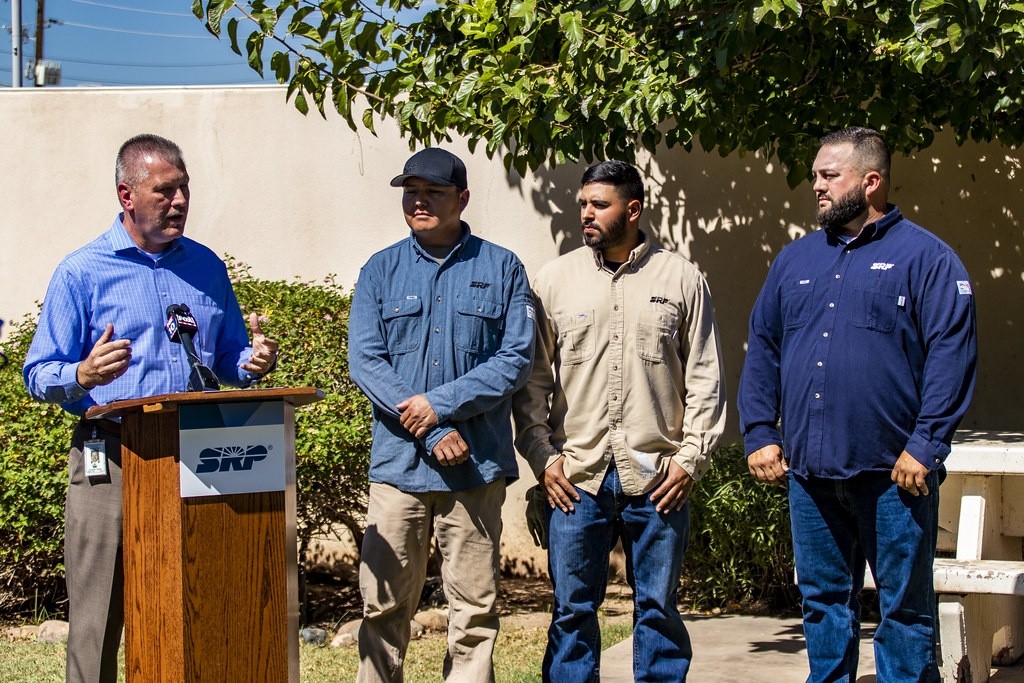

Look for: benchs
[792,559,1024,596]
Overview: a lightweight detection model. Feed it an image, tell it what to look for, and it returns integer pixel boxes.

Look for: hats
[390,148,468,188]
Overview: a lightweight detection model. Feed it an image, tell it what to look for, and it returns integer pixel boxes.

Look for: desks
[932,444,1024,683]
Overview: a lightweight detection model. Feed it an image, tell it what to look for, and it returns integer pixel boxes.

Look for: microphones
[181,303,191,316]
[173,306,185,316]
[164,303,220,393]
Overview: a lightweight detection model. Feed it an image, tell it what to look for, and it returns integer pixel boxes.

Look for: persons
[90,450,100,463]
[20,135,279,683]
[737,126,976,683]
[508,161,732,683]
[346,148,536,682]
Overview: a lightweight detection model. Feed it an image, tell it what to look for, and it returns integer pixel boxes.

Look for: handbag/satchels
[524,484,553,548]
